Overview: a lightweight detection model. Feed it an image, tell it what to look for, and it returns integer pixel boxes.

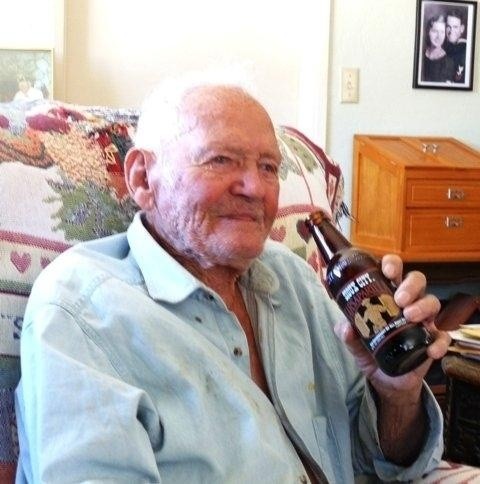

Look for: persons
[443,10,466,84]
[421,12,454,82]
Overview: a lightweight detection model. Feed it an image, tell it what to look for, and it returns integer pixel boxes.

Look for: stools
[439,353,480,466]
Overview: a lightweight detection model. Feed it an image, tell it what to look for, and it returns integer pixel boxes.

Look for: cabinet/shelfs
[352,129,480,313]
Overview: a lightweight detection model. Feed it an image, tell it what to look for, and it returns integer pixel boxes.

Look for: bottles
[304,212,433,377]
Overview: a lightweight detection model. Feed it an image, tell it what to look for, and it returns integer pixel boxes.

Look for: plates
[445,329,480,350]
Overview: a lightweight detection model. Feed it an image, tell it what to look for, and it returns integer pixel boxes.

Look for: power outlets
[340,67,361,105]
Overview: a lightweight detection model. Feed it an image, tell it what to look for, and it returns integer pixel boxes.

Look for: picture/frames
[414,0,477,93]
